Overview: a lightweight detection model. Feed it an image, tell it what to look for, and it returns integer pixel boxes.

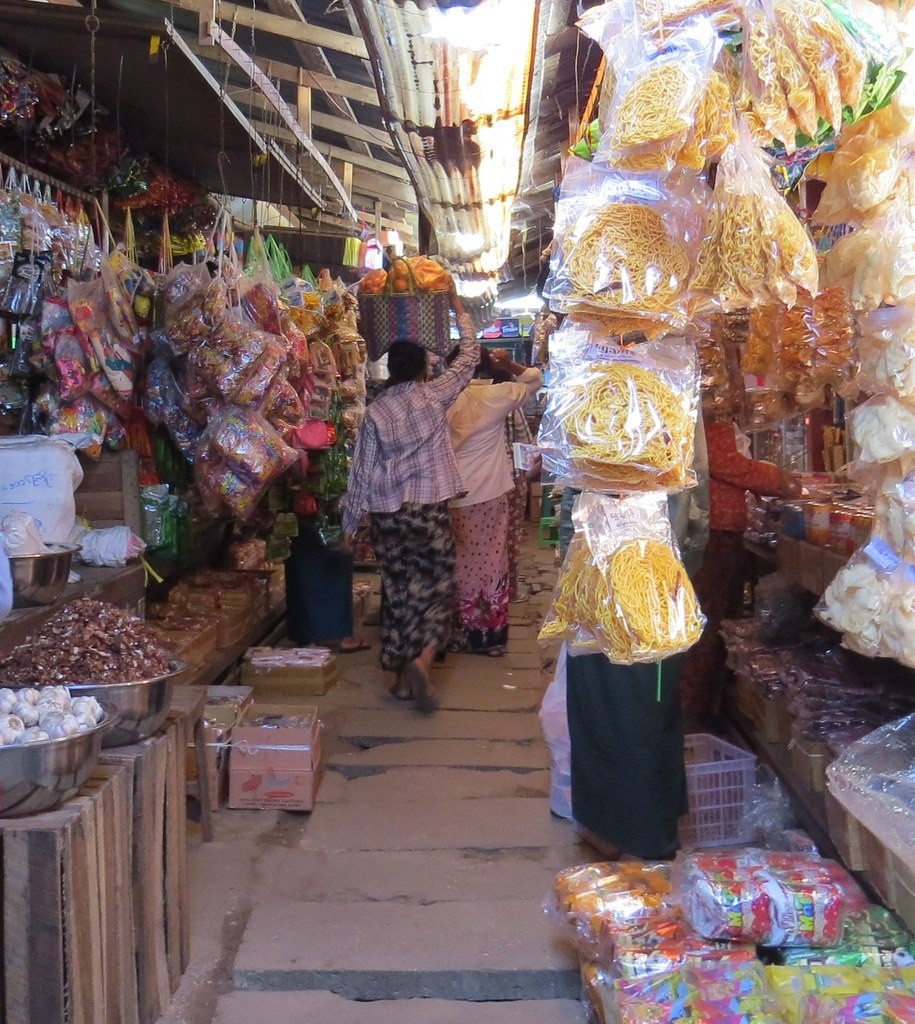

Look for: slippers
[405,661,437,712]
[390,684,413,700]
[338,640,372,653]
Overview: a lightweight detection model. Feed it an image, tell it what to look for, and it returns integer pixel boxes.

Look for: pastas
[540,0,864,664]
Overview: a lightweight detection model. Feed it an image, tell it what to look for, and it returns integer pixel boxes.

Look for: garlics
[0,683,104,747]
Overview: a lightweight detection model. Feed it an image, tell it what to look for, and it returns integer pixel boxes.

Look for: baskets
[358,248,451,362]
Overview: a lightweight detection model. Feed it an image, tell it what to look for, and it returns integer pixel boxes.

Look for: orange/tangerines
[359,256,448,293]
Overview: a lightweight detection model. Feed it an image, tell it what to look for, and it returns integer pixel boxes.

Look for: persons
[284,514,372,652]
[341,275,481,711]
[444,345,544,656]
[688,418,808,734]
[537,241,711,859]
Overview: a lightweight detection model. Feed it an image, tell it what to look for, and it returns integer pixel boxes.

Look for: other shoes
[449,644,460,652]
[489,647,504,657]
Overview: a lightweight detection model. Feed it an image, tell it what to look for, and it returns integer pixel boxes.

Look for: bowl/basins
[0,699,122,815]
[6,541,82,609]
[0,656,187,746]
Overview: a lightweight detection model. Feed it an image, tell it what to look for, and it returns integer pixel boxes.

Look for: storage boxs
[678,733,758,849]
[202,567,280,592]
[241,655,339,697]
[352,597,364,623]
[500,319,519,338]
[145,620,220,685]
[822,782,864,873]
[519,315,534,337]
[227,700,326,815]
[206,683,255,723]
[483,320,502,339]
[354,581,375,611]
[187,717,237,813]
[159,599,249,649]
[787,723,827,793]
[169,582,265,623]
[748,679,780,744]
[857,819,894,909]
[890,845,915,935]
